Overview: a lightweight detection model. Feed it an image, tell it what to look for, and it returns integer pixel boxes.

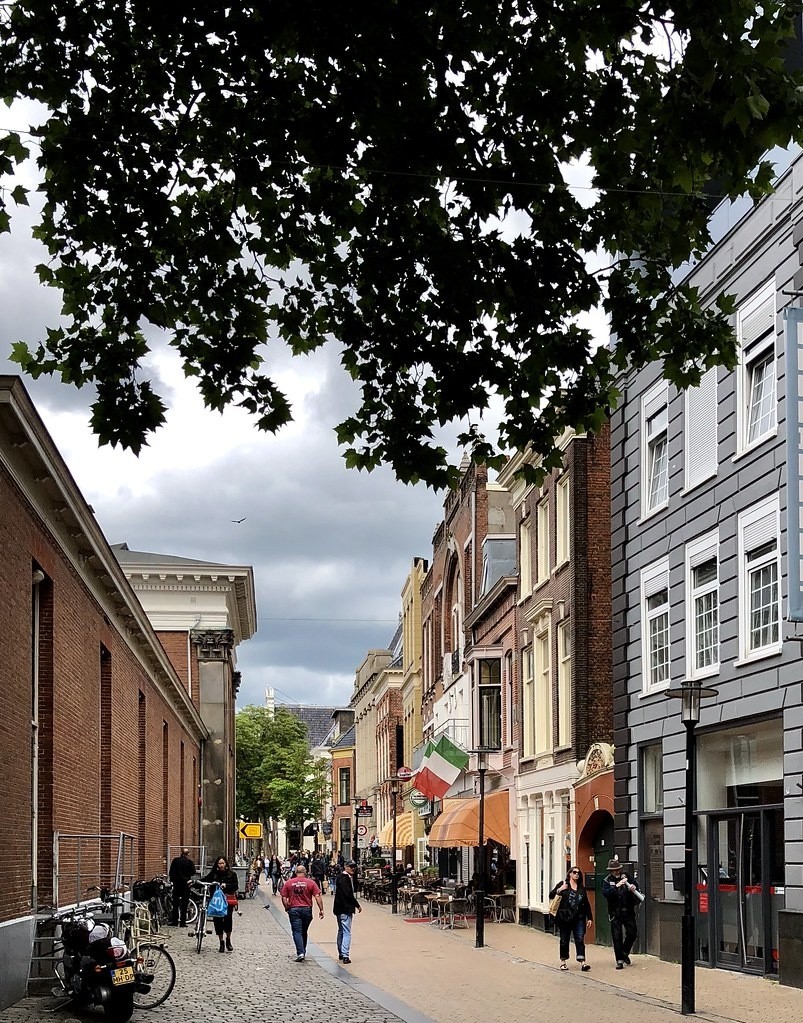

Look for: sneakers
[295,954,305,962]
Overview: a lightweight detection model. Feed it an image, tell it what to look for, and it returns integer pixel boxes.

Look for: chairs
[355,869,517,930]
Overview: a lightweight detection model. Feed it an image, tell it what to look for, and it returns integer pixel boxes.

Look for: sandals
[560,963,569,970]
[582,964,591,971]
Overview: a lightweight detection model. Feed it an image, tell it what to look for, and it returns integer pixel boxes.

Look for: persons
[466,872,505,906]
[602,859,643,969]
[281,865,325,962]
[187,856,239,953]
[235,843,344,896]
[369,834,382,859]
[374,863,413,876]
[549,866,593,971]
[167,848,196,927]
[333,860,362,964]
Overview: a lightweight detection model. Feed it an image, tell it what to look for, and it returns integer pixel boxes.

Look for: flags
[414,732,470,801]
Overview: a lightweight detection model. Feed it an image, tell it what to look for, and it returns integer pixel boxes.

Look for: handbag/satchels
[548,879,566,917]
[224,893,238,906]
[278,879,285,892]
[208,885,228,918]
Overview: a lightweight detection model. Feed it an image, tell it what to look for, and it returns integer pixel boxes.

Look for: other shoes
[167,922,188,927]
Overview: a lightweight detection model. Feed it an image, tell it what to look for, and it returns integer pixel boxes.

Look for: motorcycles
[40,902,154,1023]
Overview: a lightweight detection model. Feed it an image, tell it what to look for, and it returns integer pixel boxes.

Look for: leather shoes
[617,953,631,969]
[339,954,352,964]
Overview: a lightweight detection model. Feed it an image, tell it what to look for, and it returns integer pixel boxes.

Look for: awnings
[378,813,414,850]
[428,790,510,849]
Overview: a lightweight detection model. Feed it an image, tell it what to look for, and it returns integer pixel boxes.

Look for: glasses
[349,865,355,868]
[571,870,580,875]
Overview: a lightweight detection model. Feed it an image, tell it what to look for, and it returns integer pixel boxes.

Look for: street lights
[384,776,404,913]
[350,795,366,899]
[310,821,320,851]
[663,680,719,1015]
[467,745,499,948]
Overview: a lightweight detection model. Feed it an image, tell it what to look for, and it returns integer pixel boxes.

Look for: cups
[419,888,422,892]
[404,885,407,890]
[449,896,453,902]
[437,892,440,897]
[411,887,415,890]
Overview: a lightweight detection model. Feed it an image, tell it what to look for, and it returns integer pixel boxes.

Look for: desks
[397,887,422,916]
[425,895,449,925]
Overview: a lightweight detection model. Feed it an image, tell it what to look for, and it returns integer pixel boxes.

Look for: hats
[344,860,357,866]
[606,859,623,870]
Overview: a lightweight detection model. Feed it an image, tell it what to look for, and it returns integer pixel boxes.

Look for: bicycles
[189,880,223,954]
[246,872,260,899]
[87,874,207,924]
[104,897,176,1010]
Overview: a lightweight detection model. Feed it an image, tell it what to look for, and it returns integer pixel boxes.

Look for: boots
[218,938,234,953]
